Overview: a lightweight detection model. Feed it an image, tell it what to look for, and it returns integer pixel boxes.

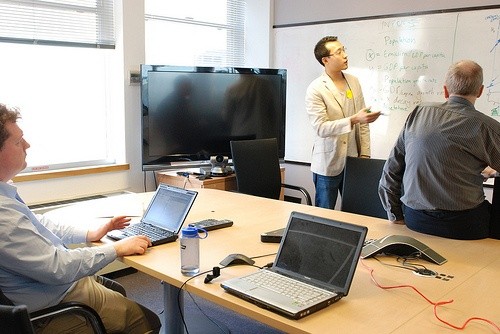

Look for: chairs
[230,138,312,206]
[341,156,404,220]
[0,290,107,334]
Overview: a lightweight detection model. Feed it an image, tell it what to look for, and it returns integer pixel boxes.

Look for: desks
[42,189,500,334]
[153,167,285,201]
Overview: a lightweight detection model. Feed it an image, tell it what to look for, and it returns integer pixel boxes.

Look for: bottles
[181,224,208,277]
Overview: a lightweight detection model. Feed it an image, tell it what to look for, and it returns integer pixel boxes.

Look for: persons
[378,60,500,240]
[304,36,381,211]
[0,105,161,334]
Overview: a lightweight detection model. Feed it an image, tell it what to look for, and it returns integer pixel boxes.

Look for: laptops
[105,183,198,247]
[219,210,368,319]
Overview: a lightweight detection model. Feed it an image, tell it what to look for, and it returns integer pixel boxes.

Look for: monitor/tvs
[140,63,287,176]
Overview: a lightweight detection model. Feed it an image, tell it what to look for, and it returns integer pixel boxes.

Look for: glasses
[324,47,347,58]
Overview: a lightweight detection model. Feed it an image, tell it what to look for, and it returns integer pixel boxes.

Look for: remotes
[188,218,233,232]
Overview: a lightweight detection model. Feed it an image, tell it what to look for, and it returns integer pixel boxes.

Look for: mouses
[219,254,256,265]
